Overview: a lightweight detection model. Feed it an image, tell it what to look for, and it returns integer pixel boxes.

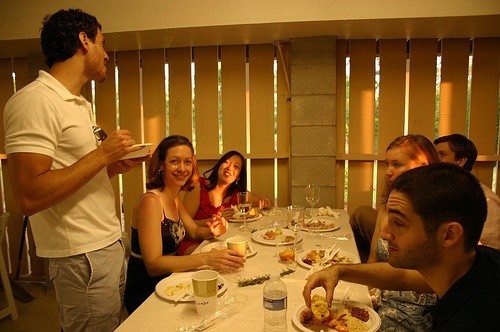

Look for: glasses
[92,125,107,142]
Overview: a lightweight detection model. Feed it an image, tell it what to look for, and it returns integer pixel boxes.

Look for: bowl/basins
[120,143,153,160]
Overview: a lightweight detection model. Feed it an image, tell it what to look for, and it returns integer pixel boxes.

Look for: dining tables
[113,208,372,331]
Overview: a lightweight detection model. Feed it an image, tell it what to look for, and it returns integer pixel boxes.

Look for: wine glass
[305,184,320,219]
[237,191,253,232]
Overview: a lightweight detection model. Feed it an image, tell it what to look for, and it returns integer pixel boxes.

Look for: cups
[191,270,220,316]
[227,237,248,256]
[259,195,270,213]
[286,205,305,231]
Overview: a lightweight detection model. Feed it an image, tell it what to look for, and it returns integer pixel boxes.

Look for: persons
[366,134,436,332]
[124,135,246,316]
[178,150,273,256]
[3,9,135,332]
[433,135,500,250]
[302,162,500,332]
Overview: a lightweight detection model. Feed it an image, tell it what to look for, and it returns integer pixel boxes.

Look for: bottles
[263,272,287,332]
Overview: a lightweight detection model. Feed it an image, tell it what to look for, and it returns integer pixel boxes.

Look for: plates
[251,228,304,246]
[201,241,257,257]
[155,273,228,302]
[302,219,340,232]
[297,249,359,269]
[228,212,262,223]
[291,297,381,332]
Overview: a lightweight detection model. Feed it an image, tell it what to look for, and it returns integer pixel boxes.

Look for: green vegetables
[238,268,295,286]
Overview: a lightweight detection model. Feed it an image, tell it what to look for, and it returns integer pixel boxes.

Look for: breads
[310,295,329,320]
[279,250,295,262]
[249,209,255,216]
